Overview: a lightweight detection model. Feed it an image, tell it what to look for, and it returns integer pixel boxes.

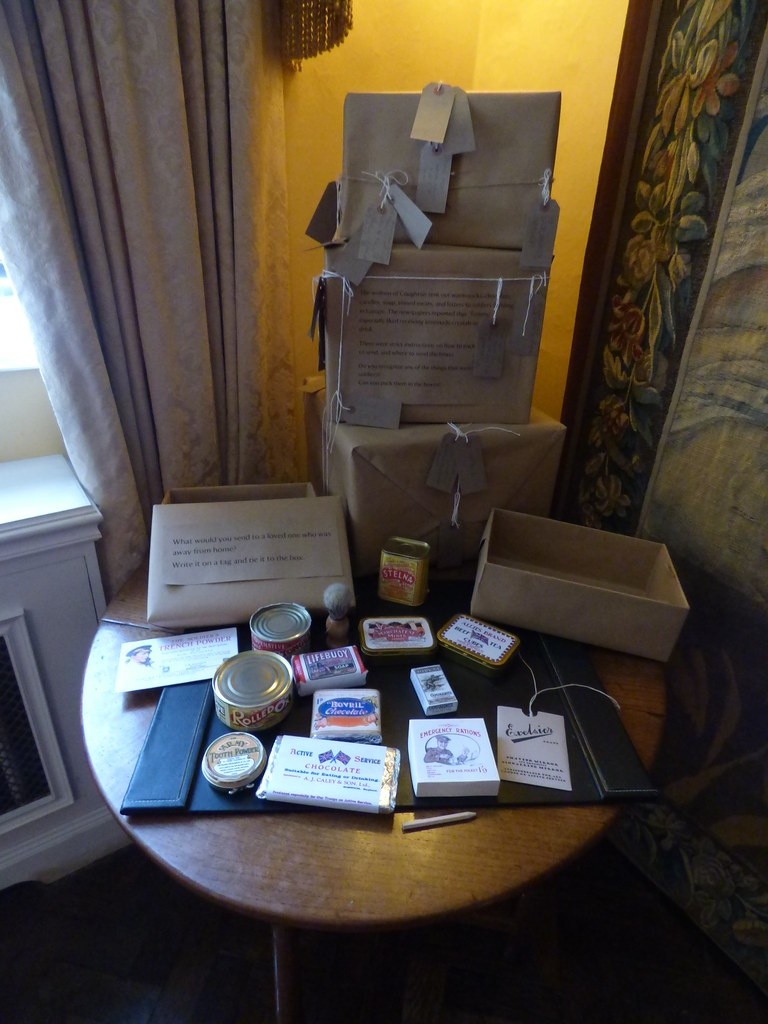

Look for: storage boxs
[201,539,555,810]
[471,509,692,665]
[409,718,499,797]
[326,405,566,583]
[150,481,356,626]
[328,250,557,428]
[333,93,564,251]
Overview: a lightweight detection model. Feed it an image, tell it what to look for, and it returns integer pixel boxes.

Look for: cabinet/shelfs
[0,451,138,889]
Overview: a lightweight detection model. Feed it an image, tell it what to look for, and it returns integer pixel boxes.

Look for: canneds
[212,650,294,733]
[249,602,312,662]
[377,536,430,606]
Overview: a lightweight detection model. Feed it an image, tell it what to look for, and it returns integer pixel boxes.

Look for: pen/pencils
[401,811,478,834]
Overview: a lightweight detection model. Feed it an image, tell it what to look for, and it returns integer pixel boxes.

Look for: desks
[82,564,703,1024]
